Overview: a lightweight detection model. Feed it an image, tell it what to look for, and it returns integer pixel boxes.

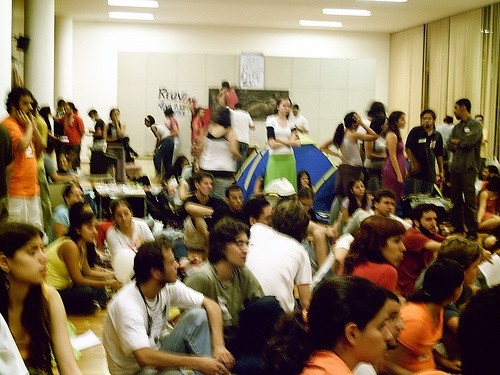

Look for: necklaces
[136,281,160,336]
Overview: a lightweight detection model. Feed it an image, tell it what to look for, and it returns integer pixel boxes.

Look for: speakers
[17,36,29,50]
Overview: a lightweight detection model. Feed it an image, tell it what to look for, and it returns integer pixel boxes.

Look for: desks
[93,181,148,222]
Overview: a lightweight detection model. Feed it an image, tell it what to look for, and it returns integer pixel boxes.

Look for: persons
[1,81,499,375]
